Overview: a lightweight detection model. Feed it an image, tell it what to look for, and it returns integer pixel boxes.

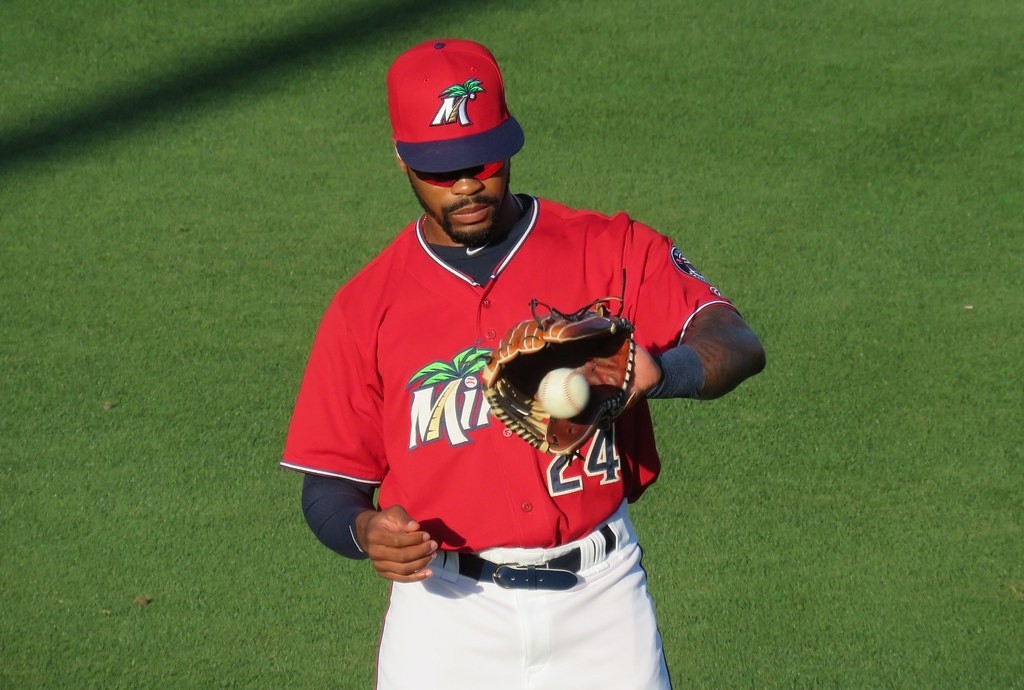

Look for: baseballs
[539,368,590,421]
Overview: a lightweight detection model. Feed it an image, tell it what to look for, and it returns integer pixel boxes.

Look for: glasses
[412,159,506,187]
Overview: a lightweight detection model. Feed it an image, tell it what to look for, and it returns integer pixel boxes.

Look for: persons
[279,38,768,690]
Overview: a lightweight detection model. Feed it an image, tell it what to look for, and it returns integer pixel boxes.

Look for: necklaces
[420,191,526,227]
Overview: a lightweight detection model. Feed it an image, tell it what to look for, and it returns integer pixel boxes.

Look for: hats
[388,37,526,173]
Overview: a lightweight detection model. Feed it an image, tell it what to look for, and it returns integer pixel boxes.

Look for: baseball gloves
[482,294,637,457]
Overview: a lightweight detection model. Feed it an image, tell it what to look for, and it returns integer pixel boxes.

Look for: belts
[442,524,616,590]
